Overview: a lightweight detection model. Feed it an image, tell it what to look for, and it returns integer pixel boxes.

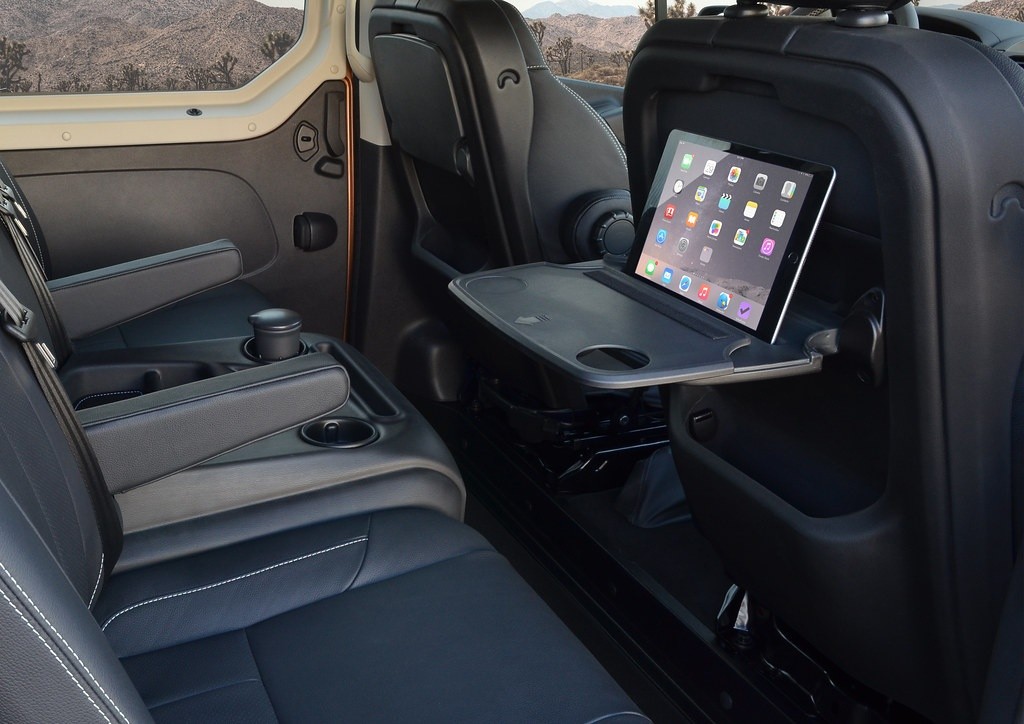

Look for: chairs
[624,0,1023,723]
[0,160,466,572]
[2,279,652,724]
[366,2,630,416]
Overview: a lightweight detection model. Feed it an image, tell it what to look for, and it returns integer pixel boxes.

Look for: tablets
[622,129,837,346]
